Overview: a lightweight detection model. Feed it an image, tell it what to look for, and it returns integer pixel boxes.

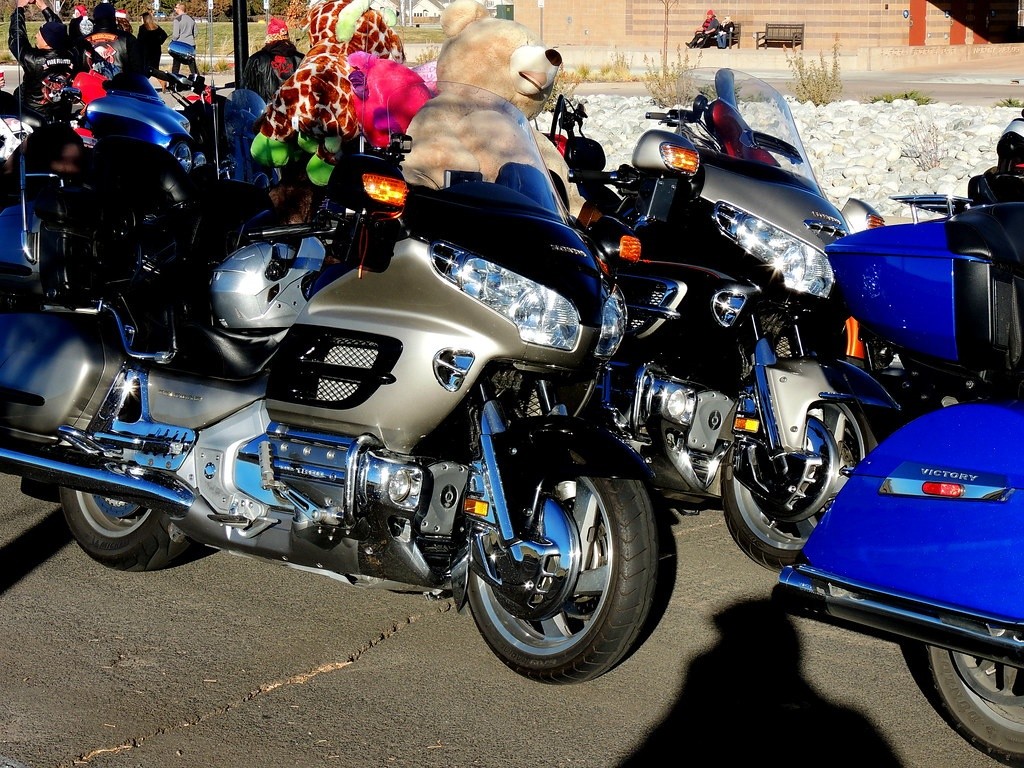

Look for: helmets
[210,242,317,329]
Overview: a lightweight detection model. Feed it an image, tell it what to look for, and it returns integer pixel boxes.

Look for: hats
[265,18,290,41]
[39,22,69,48]
[707,10,713,15]
[114,10,127,18]
[74,4,88,19]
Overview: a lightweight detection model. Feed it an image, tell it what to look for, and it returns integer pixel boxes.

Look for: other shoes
[685,42,692,48]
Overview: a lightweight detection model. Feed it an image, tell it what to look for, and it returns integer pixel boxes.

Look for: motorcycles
[2,40,237,172]
[0,86,672,685]
[543,94,1024,768]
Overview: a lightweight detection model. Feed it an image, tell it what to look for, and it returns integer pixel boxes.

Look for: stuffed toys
[251,0,437,187]
[399,0,587,221]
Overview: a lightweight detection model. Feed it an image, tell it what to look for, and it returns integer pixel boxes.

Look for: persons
[169,2,201,76]
[717,16,736,48]
[685,9,719,50]
[242,16,304,103]
[0,0,168,114]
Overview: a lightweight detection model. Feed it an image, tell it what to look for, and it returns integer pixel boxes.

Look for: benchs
[695,23,743,49]
[756,22,805,50]
